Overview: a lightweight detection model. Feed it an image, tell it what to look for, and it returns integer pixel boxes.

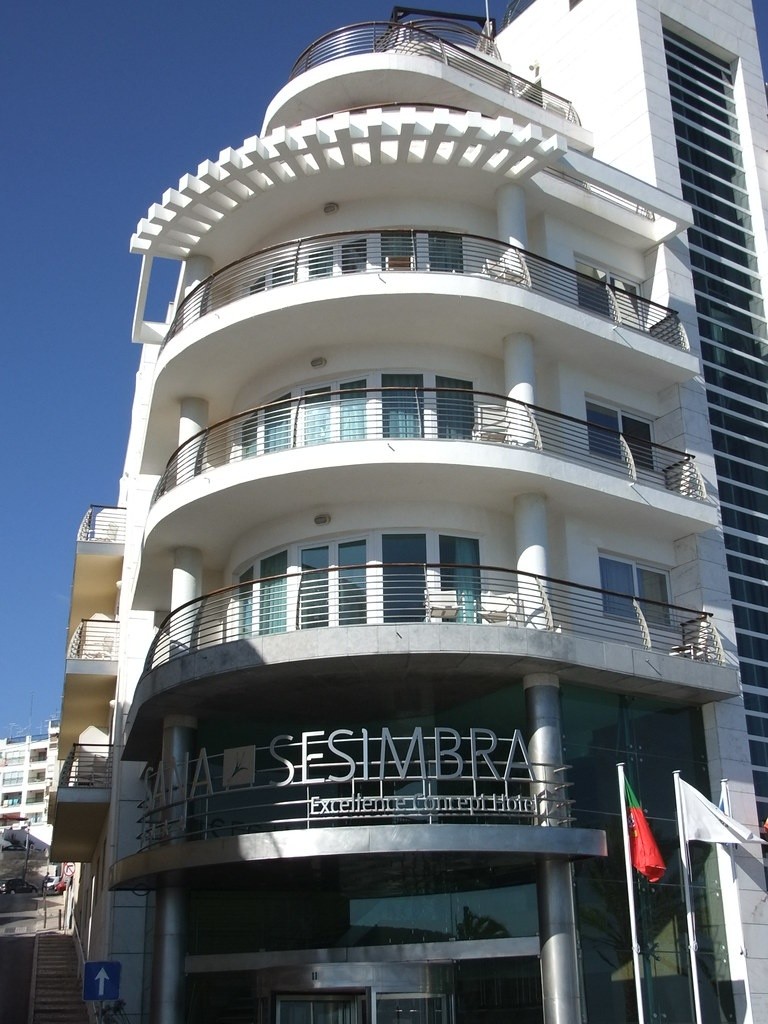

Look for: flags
[621,777,666,882]
[677,777,768,844]
[717,783,738,881]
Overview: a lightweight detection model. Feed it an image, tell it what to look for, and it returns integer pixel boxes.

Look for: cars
[2,844,26,850]
[0,877,38,895]
[38,876,66,895]
